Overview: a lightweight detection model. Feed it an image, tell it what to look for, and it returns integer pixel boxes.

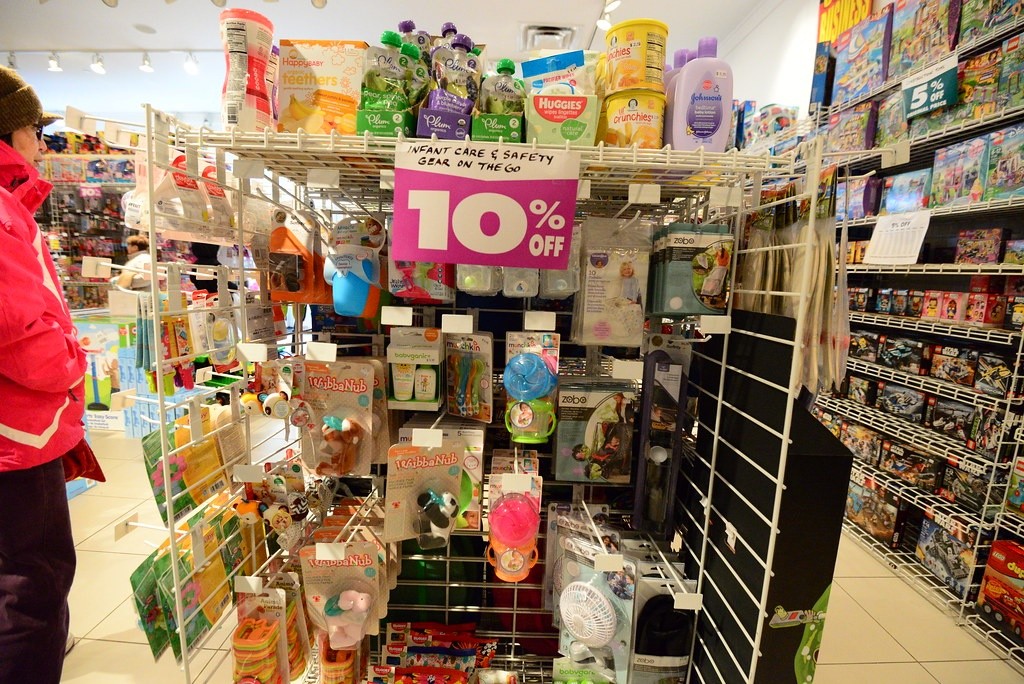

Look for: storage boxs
[356,102,420,163]
[1003,296,1024,332]
[977,540,1024,638]
[955,228,1012,264]
[921,290,944,323]
[524,94,603,180]
[833,286,878,312]
[276,37,371,139]
[968,275,1006,294]
[1004,275,1024,296]
[834,241,870,265]
[731,0,1024,222]
[844,470,910,549]
[416,92,472,140]
[1003,240,1024,264]
[874,289,893,314]
[811,330,1024,518]
[471,97,524,143]
[940,292,970,325]
[964,293,1007,328]
[906,289,925,318]
[914,506,994,603]
[891,290,908,316]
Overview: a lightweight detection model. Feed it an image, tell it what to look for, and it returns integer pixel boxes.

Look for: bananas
[289,94,326,120]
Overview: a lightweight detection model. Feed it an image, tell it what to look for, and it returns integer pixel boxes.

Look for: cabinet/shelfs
[82,102,897,684]
[738,13,1024,676]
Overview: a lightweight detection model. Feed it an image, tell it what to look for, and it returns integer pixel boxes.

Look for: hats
[0,64,43,137]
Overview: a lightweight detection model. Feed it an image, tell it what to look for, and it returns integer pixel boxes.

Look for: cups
[486,527,538,582]
[504,399,556,444]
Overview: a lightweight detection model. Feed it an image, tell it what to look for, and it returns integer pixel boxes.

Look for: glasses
[31,124,42,141]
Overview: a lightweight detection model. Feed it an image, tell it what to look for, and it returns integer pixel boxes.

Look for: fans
[559,582,618,679]
[553,553,563,597]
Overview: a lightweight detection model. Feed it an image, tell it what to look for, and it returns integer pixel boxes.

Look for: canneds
[595,18,668,148]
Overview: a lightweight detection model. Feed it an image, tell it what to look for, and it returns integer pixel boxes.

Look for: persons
[0,68,85,684]
[114,236,160,293]
